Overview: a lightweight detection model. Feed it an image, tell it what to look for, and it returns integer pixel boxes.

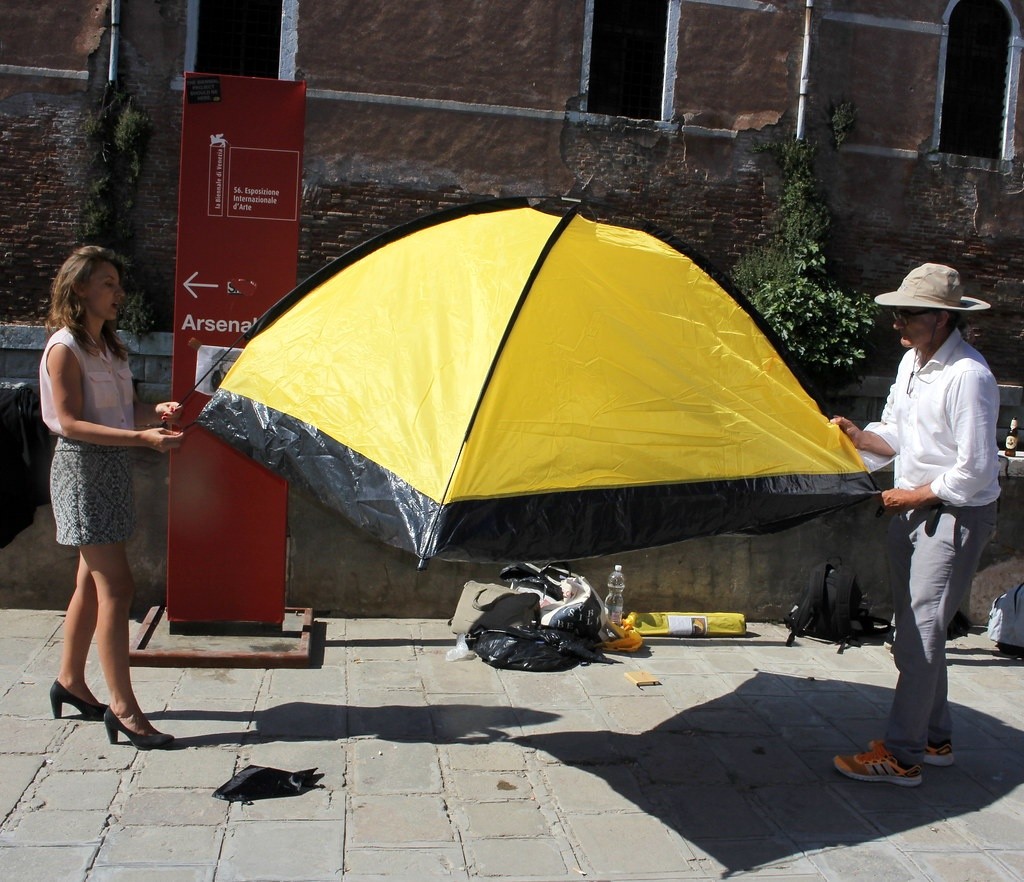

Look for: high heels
[103,703,175,751]
[50,676,110,721]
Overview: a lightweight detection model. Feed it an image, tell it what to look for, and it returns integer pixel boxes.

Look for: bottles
[1004,417,1019,457]
[604,565,625,625]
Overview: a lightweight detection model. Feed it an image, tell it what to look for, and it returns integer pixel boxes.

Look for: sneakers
[868,737,954,767]
[833,738,923,787]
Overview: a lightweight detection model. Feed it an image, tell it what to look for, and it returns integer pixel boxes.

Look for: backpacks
[783,560,891,654]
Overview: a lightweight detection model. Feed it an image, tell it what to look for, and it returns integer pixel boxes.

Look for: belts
[925,504,942,534]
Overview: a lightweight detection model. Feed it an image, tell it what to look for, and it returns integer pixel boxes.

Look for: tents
[166,193,884,571]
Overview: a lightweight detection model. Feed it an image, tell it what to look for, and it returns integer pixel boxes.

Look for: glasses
[893,309,932,325]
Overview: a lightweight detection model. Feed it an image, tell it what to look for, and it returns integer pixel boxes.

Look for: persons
[828,264,1002,788]
[38,245,185,750]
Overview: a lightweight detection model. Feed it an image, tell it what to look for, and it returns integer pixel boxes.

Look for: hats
[874,263,991,310]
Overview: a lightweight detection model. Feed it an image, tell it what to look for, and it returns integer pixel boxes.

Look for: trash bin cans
[0,383,36,549]
[23,380,51,506]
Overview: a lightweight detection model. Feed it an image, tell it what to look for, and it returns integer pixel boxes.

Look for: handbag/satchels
[987,581,1024,658]
